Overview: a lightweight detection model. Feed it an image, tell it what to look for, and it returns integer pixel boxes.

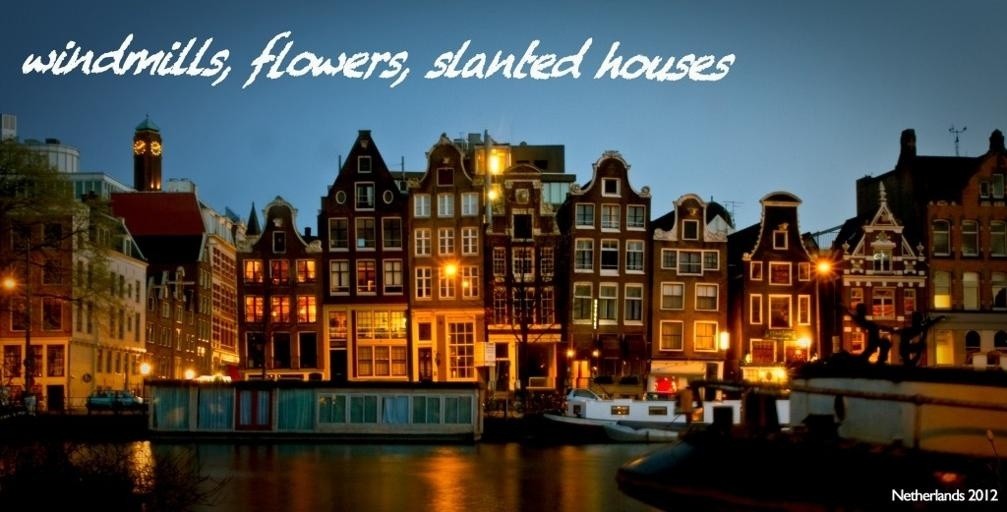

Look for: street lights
[814,259,842,354]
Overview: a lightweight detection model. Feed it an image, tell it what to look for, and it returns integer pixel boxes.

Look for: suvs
[86,389,143,412]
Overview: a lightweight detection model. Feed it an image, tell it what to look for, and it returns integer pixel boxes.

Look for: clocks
[133,139,146,155]
[150,140,163,156]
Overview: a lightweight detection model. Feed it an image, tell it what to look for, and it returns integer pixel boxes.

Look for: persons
[890,311,946,366]
[845,302,895,368]
